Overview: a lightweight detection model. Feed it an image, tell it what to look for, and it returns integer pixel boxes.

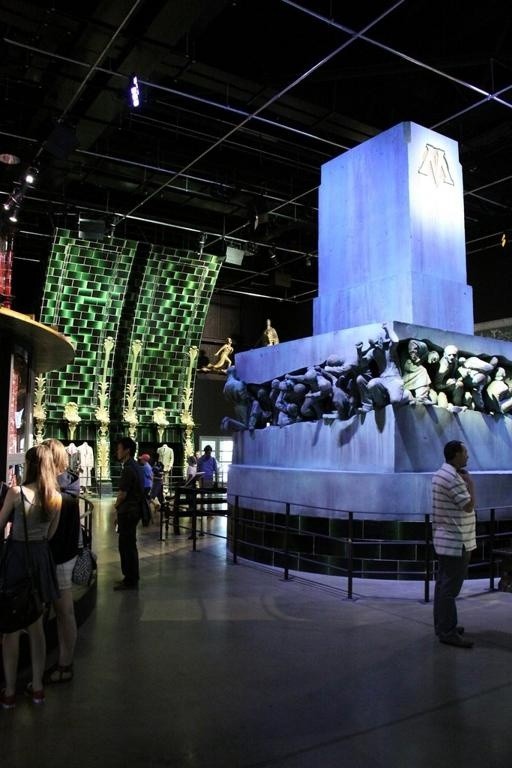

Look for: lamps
[3,160,42,228]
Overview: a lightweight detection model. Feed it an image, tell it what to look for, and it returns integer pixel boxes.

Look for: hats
[137,454,150,461]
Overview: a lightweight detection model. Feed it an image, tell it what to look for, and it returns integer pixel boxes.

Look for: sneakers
[23,679,46,704]
[439,629,475,649]
[456,626,465,635]
[4,687,21,709]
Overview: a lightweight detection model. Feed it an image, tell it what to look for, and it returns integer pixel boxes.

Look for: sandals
[43,663,74,686]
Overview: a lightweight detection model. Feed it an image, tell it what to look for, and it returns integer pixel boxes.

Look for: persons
[185,456,196,489]
[139,454,153,496]
[78,442,95,494]
[1,445,62,710]
[150,453,166,512]
[157,444,175,494]
[114,438,141,592]
[198,445,217,519]
[432,439,477,650]
[206,319,511,431]
[26,438,81,684]
[65,443,80,475]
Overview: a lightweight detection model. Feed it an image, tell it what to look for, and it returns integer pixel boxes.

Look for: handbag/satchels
[71,549,98,588]
[2,578,45,633]
[139,500,154,528]
